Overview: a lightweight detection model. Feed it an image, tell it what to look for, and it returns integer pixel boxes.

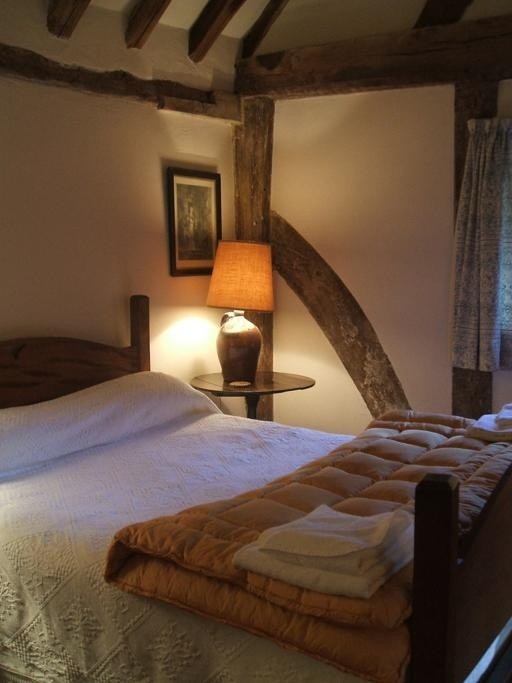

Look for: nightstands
[190,370,316,420]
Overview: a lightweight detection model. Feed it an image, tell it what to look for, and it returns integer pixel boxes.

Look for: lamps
[206,239,276,384]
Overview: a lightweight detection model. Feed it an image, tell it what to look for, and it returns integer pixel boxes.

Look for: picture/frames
[167,167,221,276]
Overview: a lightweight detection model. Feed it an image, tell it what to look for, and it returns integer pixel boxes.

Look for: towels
[231,503,415,599]
[468,402,512,442]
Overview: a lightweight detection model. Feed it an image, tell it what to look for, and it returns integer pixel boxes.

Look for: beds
[1,293,512,682]
